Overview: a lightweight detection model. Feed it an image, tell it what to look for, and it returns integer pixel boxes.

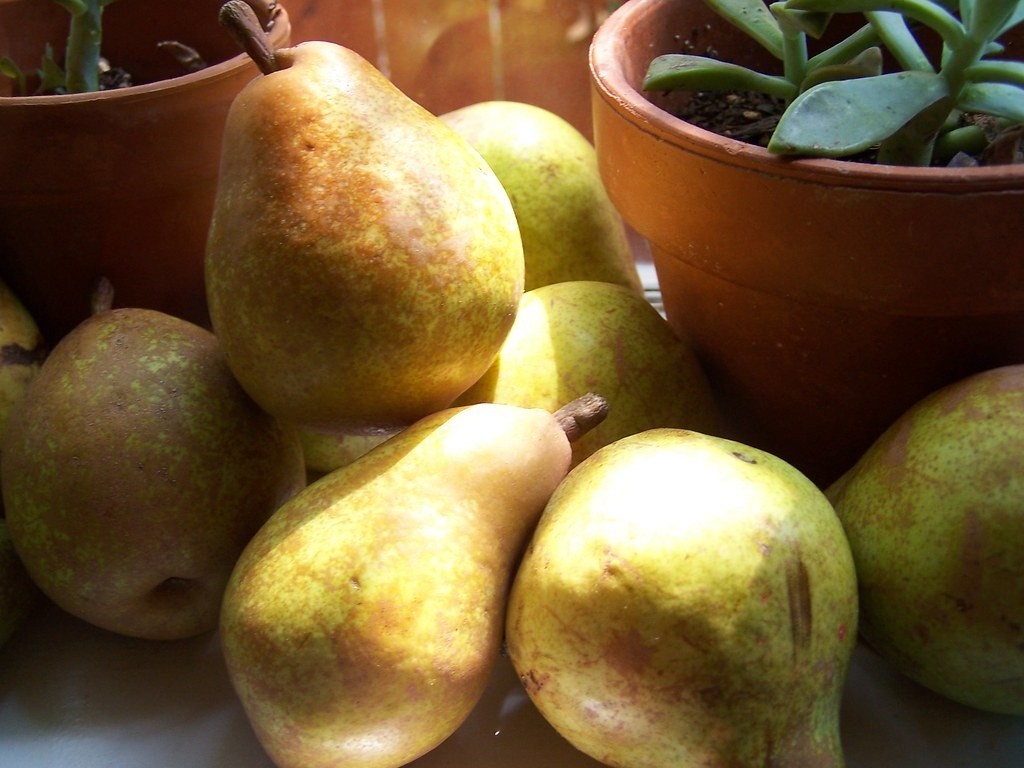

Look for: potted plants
[0,0,294,344]
[588,0,1024,484]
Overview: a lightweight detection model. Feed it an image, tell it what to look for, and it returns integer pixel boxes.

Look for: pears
[0,1,1024,768]
